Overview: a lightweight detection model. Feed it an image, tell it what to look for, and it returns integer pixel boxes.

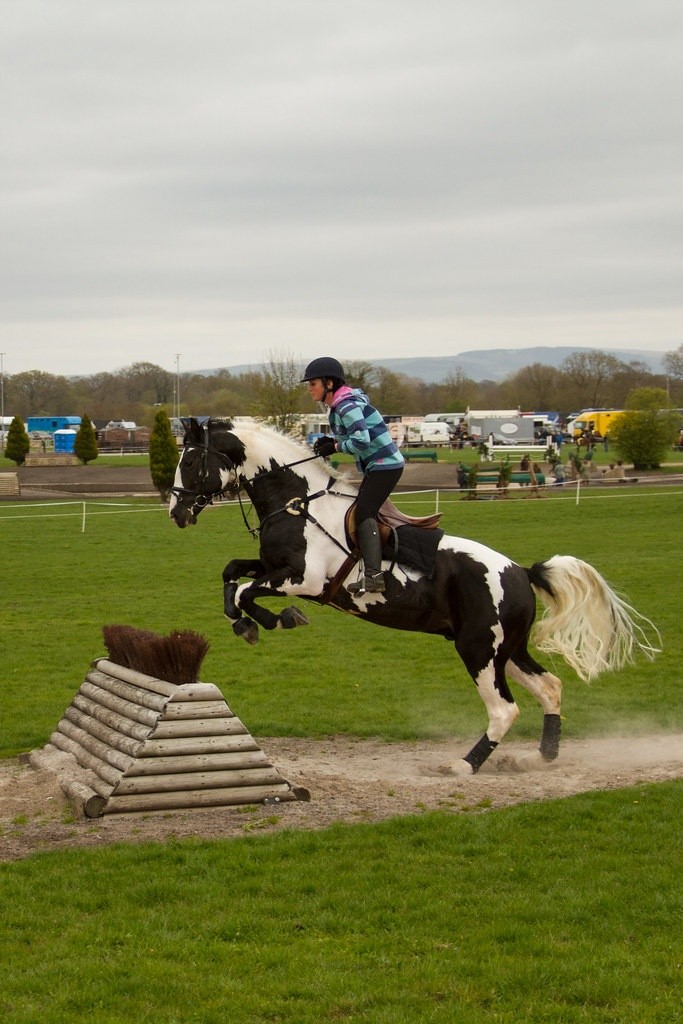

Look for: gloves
[314,436,338,458]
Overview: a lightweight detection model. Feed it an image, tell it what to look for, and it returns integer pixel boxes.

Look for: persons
[298,356,405,592]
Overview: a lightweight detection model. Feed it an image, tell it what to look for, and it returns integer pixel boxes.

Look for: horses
[163,415,666,778]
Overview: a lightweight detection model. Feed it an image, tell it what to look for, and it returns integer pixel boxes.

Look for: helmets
[300,357,346,383]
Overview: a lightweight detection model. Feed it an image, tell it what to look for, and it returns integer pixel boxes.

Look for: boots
[347,517,386,592]
